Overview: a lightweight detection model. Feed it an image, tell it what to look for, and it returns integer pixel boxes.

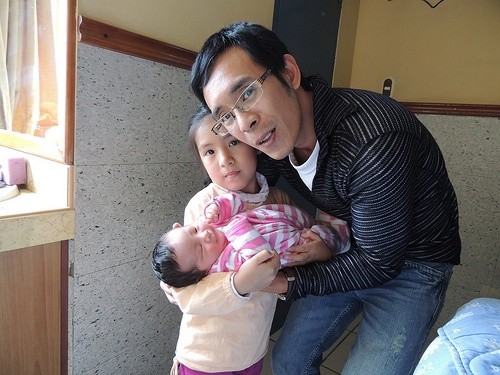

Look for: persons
[151,203,350,290]
[160,101,337,375]
[189,21,463,375]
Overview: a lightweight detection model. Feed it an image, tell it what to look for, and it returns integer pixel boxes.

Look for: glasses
[211,61,278,137]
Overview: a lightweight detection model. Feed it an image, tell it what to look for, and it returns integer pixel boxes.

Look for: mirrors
[0,0,76,166]
